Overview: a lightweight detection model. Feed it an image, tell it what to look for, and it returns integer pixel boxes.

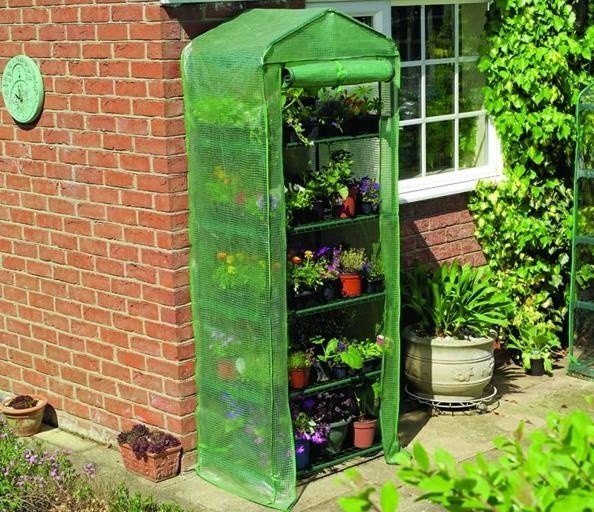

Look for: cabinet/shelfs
[180,7,403,512]
[567,84,594,379]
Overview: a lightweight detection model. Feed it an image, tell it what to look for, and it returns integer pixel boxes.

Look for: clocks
[1,55,45,124]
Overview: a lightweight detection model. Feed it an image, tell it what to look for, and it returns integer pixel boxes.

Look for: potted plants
[118,425,180,483]
[404,259,513,402]
[0,395,47,437]
